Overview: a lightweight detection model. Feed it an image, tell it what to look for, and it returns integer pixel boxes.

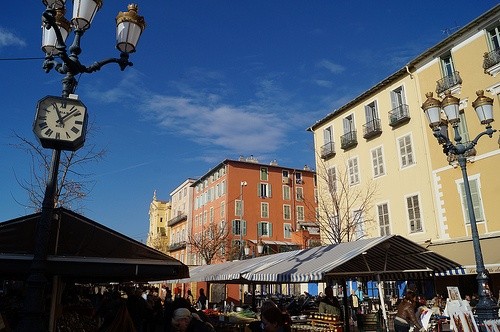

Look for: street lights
[420,87,500,332]
[19,1,148,332]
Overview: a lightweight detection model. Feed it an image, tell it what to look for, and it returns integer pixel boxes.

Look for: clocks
[32,94,88,152]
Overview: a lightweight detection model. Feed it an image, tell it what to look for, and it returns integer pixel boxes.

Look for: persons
[0,279,500,332]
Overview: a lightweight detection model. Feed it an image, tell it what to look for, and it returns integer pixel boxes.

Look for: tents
[147,233,466,332]
